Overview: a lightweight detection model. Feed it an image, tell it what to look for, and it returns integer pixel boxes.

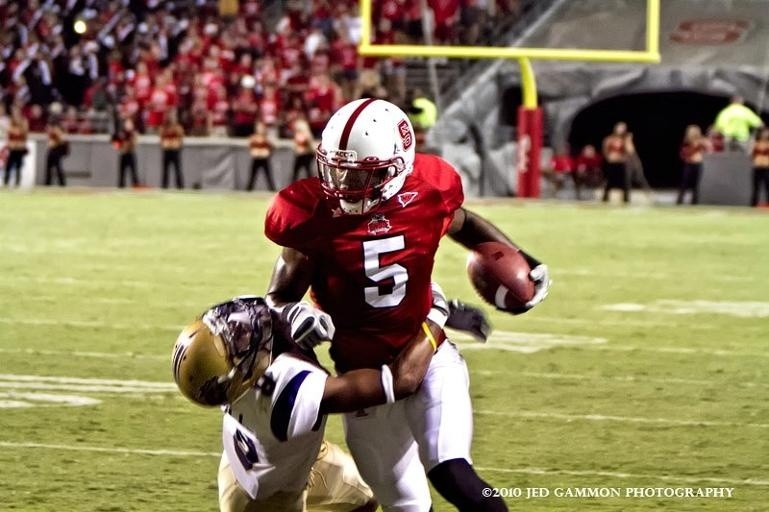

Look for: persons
[675,124,710,204]
[601,122,635,203]
[290,119,316,180]
[4,106,28,184]
[713,96,764,138]
[0,103,10,169]
[413,122,429,150]
[264,94,553,512]
[41,121,68,186]
[158,118,184,189]
[245,122,275,191]
[750,128,768,206]
[706,128,726,150]
[541,144,573,195]
[0,1,523,139]
[111,119,140,186]
[572,146,604,202]
[170,281,449,512]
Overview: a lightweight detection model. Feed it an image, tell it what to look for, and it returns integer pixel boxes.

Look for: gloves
[446,295,492,342]
[286,300,336,349]
[425,281,451,328]
[528,261,553,308]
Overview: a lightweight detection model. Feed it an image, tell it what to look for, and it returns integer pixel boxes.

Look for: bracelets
[422,323,437,352]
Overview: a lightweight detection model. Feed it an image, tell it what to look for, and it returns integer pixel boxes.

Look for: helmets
[313,97,417,216]
[169,295,274,410]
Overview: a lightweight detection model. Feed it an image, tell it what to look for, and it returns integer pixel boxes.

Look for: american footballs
[467,241,533,313]
